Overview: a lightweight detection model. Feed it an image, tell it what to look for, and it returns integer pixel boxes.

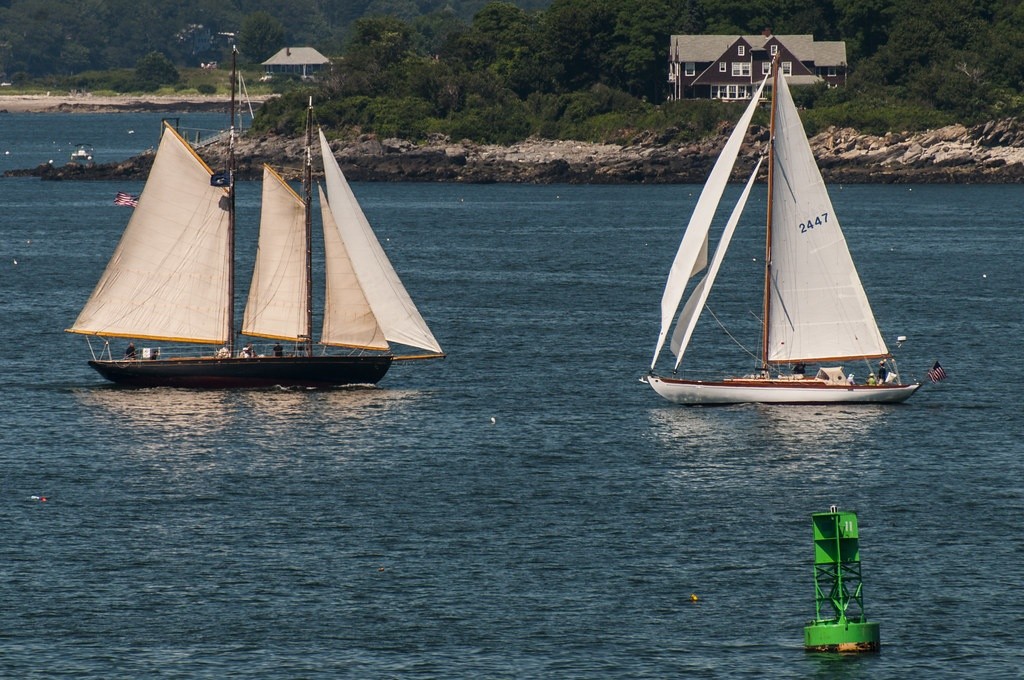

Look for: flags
[928,362,948,383]
[114,191,140,208]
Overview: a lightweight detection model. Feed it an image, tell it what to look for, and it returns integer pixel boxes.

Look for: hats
[879,361,884,364]
[847,374,855,379]
[869,373,874,376]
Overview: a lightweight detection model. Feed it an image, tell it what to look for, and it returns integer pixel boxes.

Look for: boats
[72,143,93,161]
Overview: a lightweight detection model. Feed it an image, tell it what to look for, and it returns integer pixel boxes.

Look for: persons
[273,342,283,356]
[866,373,877,386]
[240,345,258,358]
[126,341,136,360]
[794,362,806,375]
[879,361,886,384]
[150,351,158,360]
[847,374,856,385]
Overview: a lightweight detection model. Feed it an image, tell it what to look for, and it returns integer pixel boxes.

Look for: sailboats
[66,46,450,390]
[643,48,922,404]
